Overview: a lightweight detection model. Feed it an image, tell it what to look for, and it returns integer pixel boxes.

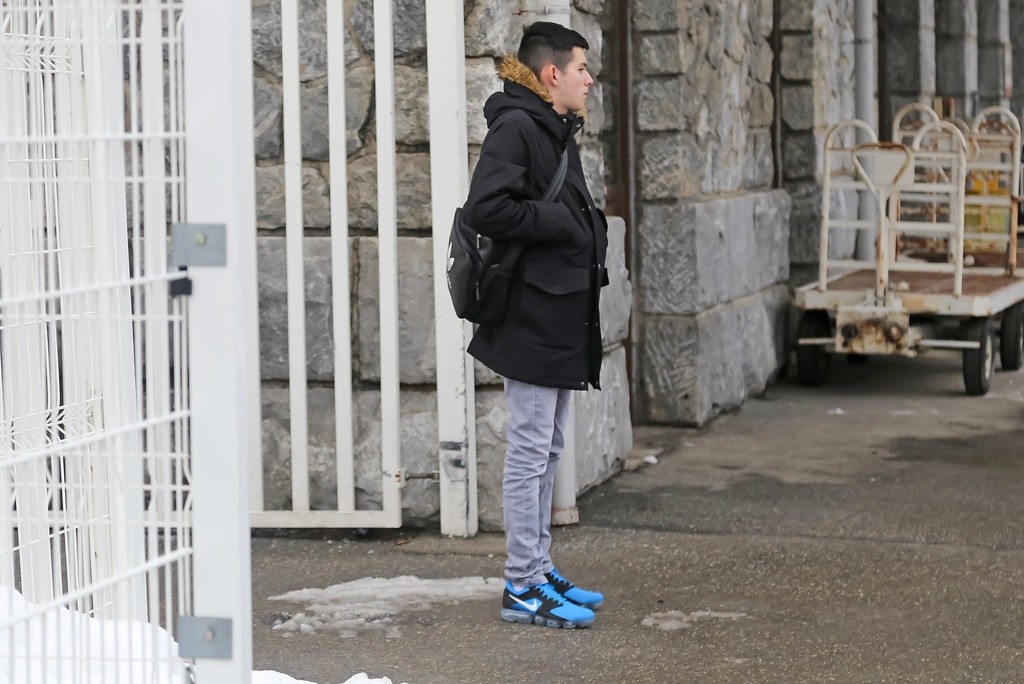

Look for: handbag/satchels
[445,208,513,325]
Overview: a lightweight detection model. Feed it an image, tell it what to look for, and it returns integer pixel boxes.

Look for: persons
[465,22,611,631]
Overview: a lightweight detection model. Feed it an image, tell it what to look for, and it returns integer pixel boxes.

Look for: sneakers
[545,569,604,610]
[501,581,597,629]
